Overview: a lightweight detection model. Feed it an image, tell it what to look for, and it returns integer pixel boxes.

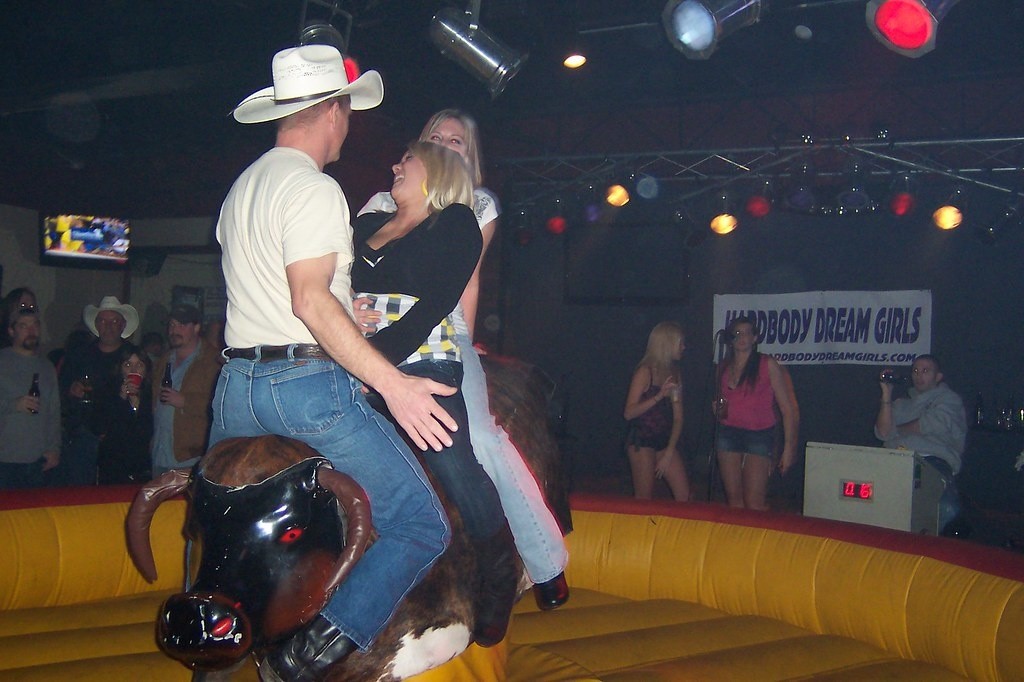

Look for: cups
[713,397,728,418]
[126,373,143,395]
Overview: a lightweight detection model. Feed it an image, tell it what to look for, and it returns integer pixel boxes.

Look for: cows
[122,348,575,682]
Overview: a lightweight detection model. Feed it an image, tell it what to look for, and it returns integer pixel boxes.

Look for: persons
[184,46,571,682]
[712,316,800,512]
[0,286,227,488]
[624,320,694,502]
[873,354,967,532]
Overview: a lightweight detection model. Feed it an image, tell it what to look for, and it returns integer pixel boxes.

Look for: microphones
[722,329,738,342]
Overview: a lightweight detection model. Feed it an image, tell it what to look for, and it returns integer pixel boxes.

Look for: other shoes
[534,571,570,611]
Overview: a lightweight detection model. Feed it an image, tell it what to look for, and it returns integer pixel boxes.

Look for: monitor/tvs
[39,213,132,271]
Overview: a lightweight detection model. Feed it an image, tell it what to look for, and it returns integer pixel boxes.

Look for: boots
[469,516,522,647]
[259,613,359,682]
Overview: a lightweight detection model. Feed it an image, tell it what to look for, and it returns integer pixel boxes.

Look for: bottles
[29,374,40,413]
[161,362,173,402]
[975,392,984,426]
[669,375,679,403]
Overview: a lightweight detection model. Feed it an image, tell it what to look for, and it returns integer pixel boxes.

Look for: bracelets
[654,392,664,404]
[880,400,892,405]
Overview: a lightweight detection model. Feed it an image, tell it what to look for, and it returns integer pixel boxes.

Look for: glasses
[14,301,37,312]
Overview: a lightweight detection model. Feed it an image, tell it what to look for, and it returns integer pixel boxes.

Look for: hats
[233,44,385,124]
[162,302,203,327]
[81,295,140,339]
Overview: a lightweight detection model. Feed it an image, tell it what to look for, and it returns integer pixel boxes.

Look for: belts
[227,344,329,362]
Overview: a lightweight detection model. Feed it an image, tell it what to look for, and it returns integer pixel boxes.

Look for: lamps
[671,174,778,251]
[427,0,533,101]
[661,1,764,60]
[295,0,360,83]
[866,0,958,59]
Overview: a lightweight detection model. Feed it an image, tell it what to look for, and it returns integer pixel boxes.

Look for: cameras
[880,372,912,383]
